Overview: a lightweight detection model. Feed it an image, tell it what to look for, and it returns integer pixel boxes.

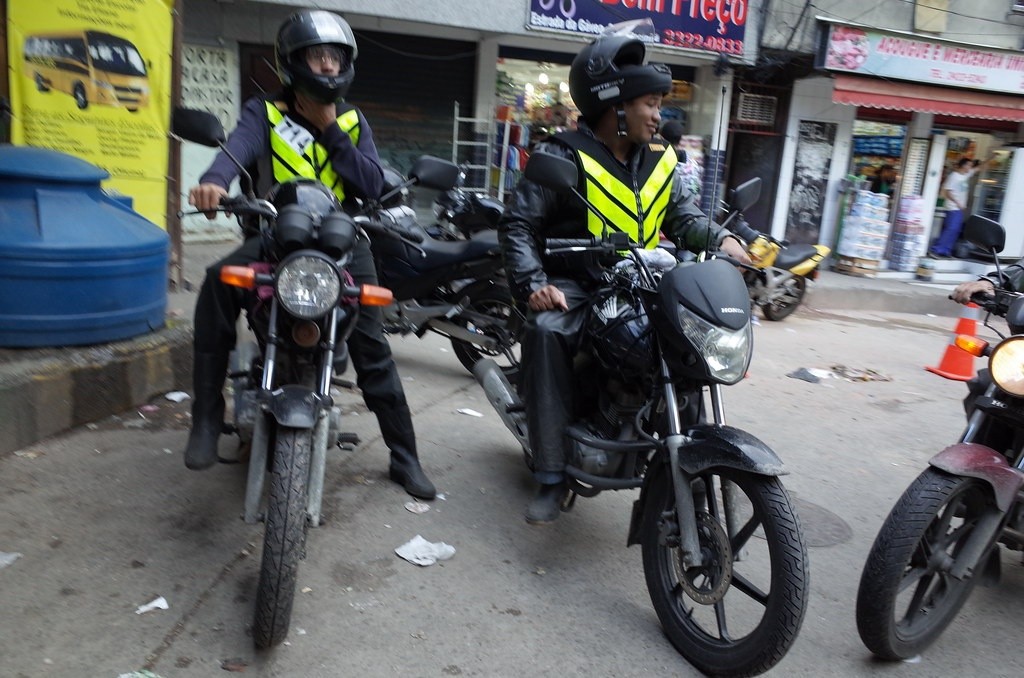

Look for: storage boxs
[835,255,881,278]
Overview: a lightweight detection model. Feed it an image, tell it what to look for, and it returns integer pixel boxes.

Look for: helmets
[276,10,357,104]
[570,37,672,114]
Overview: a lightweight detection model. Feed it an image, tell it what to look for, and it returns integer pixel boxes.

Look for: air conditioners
[730,91,779,125]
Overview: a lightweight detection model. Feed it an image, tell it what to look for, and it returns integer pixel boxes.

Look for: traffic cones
[923,294,980,382]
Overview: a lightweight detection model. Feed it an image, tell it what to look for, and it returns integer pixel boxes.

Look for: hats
[873,164,894,174]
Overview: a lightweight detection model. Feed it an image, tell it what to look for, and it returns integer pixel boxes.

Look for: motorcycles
[855,216,1024,661]
[369,185,528,371]
[165,105,462,652]
[697,183,833,324]
[473,152,809,678]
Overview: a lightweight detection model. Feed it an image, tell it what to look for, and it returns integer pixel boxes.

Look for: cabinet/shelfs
[490,114,525,207]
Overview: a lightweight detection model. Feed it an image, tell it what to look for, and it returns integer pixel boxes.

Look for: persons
[927,158,996,259]
[498,18,752,525]
[952,257,1024,449]
[184,8,437,500]
[871,166,896,197]
[662,120,703,204]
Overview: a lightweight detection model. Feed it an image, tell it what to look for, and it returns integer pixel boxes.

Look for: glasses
[306,46,341,66]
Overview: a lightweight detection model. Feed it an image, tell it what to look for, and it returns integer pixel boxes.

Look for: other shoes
[524,484,570,526]
[183,393,225,470]
[388,460,436,501]
[928,251,944,260]
